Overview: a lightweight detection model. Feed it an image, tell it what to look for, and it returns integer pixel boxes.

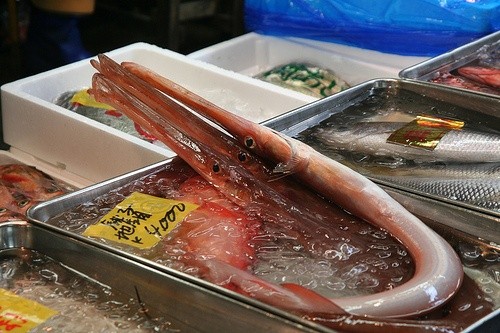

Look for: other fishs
[0,50,500,333]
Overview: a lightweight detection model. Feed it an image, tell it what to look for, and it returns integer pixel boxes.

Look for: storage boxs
[185,0,500,110]
[0,44,321,184]
